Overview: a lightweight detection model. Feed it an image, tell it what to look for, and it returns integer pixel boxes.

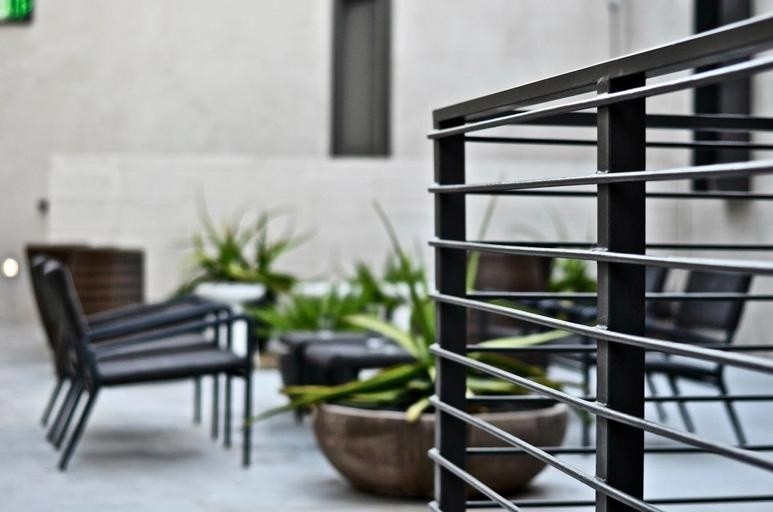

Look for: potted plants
[169,195,294,362]
[241,310,591,498]
